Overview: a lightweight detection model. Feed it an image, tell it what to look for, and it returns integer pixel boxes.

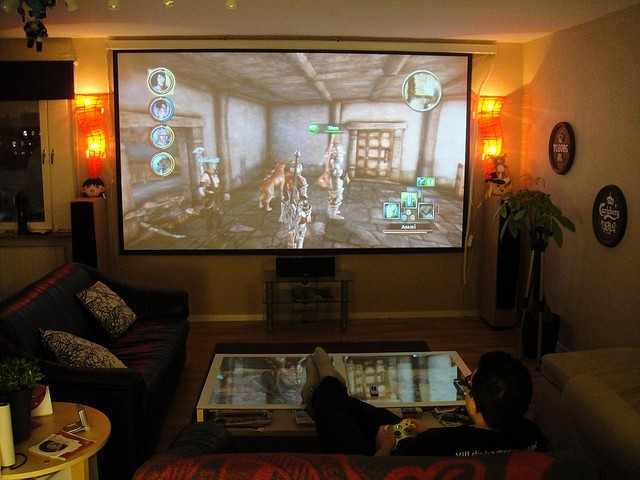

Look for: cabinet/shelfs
[262,273,352,333]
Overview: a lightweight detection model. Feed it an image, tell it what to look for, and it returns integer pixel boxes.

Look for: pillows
[38,328,128,368]
[75,280,138,339]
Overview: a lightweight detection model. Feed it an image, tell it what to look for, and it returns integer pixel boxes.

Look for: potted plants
[0,358,46,444]
[493,188,576,361]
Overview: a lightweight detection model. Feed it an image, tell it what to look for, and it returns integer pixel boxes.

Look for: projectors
[15,1,54,52]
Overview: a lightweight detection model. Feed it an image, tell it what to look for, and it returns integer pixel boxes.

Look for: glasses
[464,373,474,391]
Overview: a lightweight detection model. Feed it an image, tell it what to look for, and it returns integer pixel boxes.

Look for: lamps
[479,97,505,180]
[75,91,110,178]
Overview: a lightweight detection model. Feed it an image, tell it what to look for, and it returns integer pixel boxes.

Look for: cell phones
[453,378,467,393]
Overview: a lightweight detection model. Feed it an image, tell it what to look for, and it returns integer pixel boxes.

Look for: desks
[0,402,112,480]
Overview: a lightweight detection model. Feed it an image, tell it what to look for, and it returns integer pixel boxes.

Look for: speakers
[69,198,110,268]
[482,195,530,328]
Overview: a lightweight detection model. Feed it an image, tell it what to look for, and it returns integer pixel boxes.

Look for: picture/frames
[592,185,626,248]
[547,123,576,175]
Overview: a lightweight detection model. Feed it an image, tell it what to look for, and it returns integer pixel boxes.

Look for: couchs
[132,424,601,480]
[1,264,189,480]
[540,345,640,479]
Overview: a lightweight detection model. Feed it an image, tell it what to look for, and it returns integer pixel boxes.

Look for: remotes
[370,385,378,396]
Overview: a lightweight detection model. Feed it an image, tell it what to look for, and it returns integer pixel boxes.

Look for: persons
[300,346,556,458]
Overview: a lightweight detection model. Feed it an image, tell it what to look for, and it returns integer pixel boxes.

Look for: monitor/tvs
[0,61,74,102]
